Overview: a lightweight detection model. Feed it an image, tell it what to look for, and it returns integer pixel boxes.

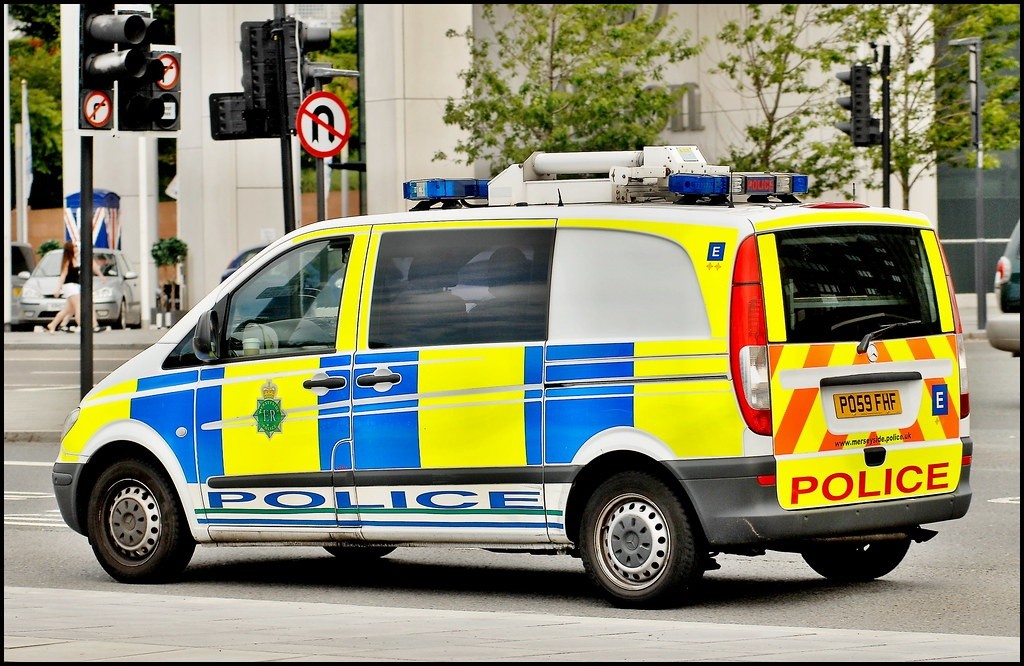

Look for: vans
[48,139,976,609]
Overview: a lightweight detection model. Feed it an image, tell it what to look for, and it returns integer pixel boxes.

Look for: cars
[216,240,404,323]
[3,241,36,333]
[17,245,142,331]
[986,215,1021,360]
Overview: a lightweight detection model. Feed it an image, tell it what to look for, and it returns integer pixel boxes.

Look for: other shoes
[76,326,80,332]
[93,326,106,332]
[58,326,74,333]
[44,328,57,335]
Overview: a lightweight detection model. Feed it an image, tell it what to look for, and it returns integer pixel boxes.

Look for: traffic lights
[832,65,872,147]
[112,3,185,134]
[75,3,148,136]
[281,16,336,136]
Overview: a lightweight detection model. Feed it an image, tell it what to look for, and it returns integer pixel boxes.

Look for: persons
[47,240,107,335]
[470,245,530,340]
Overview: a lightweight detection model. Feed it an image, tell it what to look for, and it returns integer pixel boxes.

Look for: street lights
[947,35,988,330]
[309,66,361,284]
